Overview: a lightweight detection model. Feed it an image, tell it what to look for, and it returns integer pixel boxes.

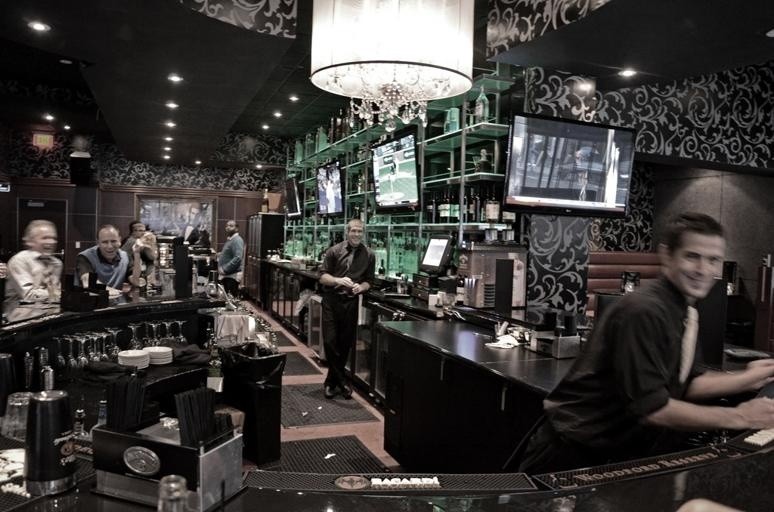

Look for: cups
[23,389,76,496]
[4,393,33,441]
[494,324,505,338]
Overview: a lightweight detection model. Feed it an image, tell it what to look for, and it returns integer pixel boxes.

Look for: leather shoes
[324,384,337,400]
[341,383,354,400]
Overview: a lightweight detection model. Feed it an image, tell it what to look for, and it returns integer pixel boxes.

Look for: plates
[726,348,769,359]
[117,345,173,371]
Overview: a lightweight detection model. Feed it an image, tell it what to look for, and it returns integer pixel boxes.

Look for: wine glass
[127,320,190,352]
[53,328,122,372]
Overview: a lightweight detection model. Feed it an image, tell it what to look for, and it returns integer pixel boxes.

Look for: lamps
[311,0,475,105]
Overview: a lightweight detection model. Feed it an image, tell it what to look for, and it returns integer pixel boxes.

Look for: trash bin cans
[219,342,287,465]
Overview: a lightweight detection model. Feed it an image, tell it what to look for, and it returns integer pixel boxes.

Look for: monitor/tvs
[286,178,302,218]
[371,125,421,214]
[419,235,455,274]
[315,160,345,219]
[501,112,637,218]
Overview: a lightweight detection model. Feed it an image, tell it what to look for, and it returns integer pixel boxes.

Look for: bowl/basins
[507,328,530,341]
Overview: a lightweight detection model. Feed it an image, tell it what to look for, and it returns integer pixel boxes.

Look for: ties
[677,305,701,385]
[346,247,357,267]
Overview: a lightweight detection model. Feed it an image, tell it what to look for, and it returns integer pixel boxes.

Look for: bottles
[439,107,459,134]
[192,266,219,297]
[267,249,280,260]
[152,474,191,511]
[470,85,490,126]
[455,274,467,306]
[140,272,148,293]
[425,186,500,223]
[226,291,279,356]
[205,327,222,376]
[0,343,50,417]
[76,399,107,439]
[282,105,417,275]
[261,188,270,213]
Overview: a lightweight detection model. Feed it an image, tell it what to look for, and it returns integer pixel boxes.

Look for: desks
[376,317,585,474]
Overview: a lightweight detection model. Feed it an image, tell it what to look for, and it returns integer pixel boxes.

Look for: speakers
[70,156,91,187]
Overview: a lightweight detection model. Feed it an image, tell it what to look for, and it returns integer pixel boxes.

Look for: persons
[516,211,774,490]
[182,205,201,245]
[317,219,375,399]
[120,219,245,299]
[74,223,142,297]
[386,155,400,194]
[3,220,64,316]
[322,180,337,213]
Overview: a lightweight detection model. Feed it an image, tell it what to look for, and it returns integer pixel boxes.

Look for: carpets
[261,435,393,473]
[281,351,323,376]
[280,382,380,428]
[266,330,297,347]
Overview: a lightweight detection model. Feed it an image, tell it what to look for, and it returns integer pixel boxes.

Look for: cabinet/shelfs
[243,213,287,300]
